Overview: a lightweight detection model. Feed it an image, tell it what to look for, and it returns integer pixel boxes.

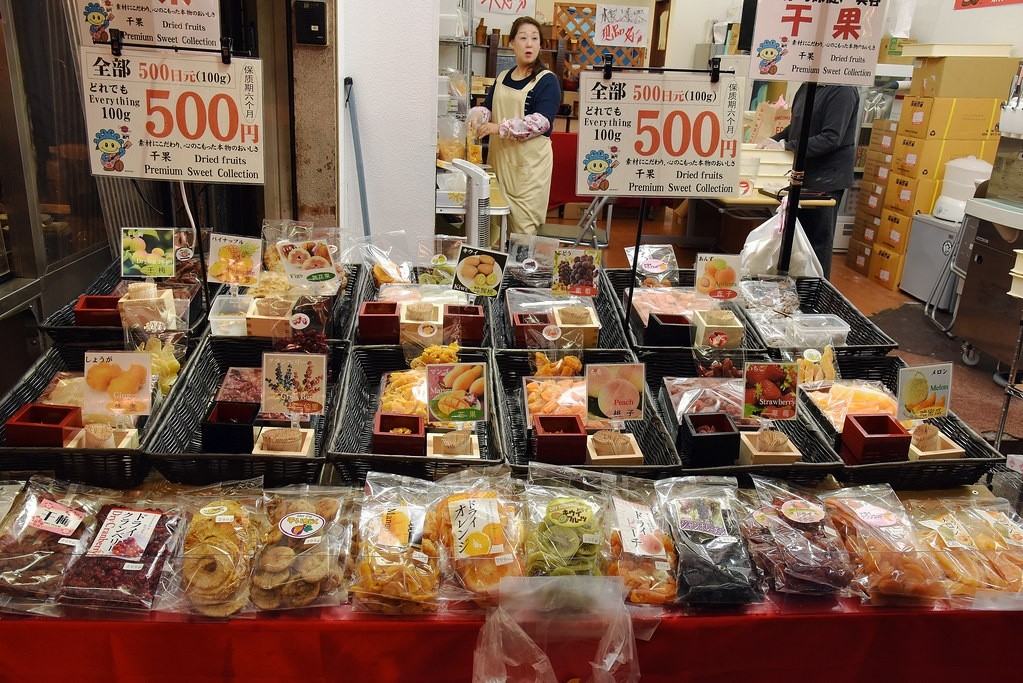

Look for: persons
[758,82,858,280]
[467,16,560,235]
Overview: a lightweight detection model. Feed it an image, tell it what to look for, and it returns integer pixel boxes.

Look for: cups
[492,29,510,47]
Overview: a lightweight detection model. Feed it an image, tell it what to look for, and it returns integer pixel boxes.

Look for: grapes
[557,254,597,288]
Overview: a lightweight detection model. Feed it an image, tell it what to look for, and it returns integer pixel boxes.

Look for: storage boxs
[548,200,604,222]
[740,141,796,198]
[470,75,496,106]
[845,37,1019,293]
[663,206,701,249]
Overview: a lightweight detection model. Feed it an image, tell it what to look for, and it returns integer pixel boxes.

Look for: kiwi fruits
[526,498,603,609]
[461,256,498,286]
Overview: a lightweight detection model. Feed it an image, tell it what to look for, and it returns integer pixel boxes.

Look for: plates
[458,255,503,289]
[430,391,482,422]
[598,380,640,417]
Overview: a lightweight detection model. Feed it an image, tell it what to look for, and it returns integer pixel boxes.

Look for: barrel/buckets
[739,157,762,197]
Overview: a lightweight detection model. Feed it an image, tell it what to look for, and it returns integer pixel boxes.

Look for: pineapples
[209,241,258,287]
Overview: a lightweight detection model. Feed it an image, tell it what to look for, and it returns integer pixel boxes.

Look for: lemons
[86,363,146,401]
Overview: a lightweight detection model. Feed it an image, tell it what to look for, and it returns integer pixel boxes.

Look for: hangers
[91,28,253,65]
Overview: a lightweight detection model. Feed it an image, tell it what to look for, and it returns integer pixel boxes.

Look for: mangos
[438,363,483,417]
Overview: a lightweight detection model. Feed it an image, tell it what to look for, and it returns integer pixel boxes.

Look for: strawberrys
[743,363,797,420]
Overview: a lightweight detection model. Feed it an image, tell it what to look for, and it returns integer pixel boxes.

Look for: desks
[717,187,836,281]
[0,482,1023,683]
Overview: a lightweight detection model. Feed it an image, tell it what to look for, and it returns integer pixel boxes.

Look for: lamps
[567,7,577,14]
[582,7,592,16]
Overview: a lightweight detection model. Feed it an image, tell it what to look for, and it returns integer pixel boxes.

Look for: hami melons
[902,377,945,417]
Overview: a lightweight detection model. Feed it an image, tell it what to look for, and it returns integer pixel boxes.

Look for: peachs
[588,365,643,420]
[121,236,164,266]
[280,241,332,272]
[697,256,736,293]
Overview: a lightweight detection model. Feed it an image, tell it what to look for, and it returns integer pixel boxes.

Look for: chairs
[923,179,991,340]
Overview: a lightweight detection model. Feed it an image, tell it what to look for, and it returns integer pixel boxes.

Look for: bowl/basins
[208,293,254,336]
[786,313,850,345]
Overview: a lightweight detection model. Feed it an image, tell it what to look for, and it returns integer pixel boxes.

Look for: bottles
[476,18,487,45]
